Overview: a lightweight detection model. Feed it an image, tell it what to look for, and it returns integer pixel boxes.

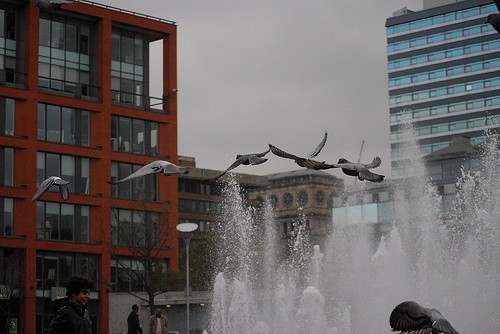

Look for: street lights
[176,221,199,334]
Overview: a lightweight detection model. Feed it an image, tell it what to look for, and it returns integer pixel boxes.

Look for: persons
[127,303,143,334]
[47,276,92,334]
[149,309,168,334]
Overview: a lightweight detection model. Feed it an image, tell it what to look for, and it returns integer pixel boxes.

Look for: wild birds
[103,159,195,186]
[200,146,271,182]
[267,127,329,170]
[30,175,72,202]
[310,153,386,184]
